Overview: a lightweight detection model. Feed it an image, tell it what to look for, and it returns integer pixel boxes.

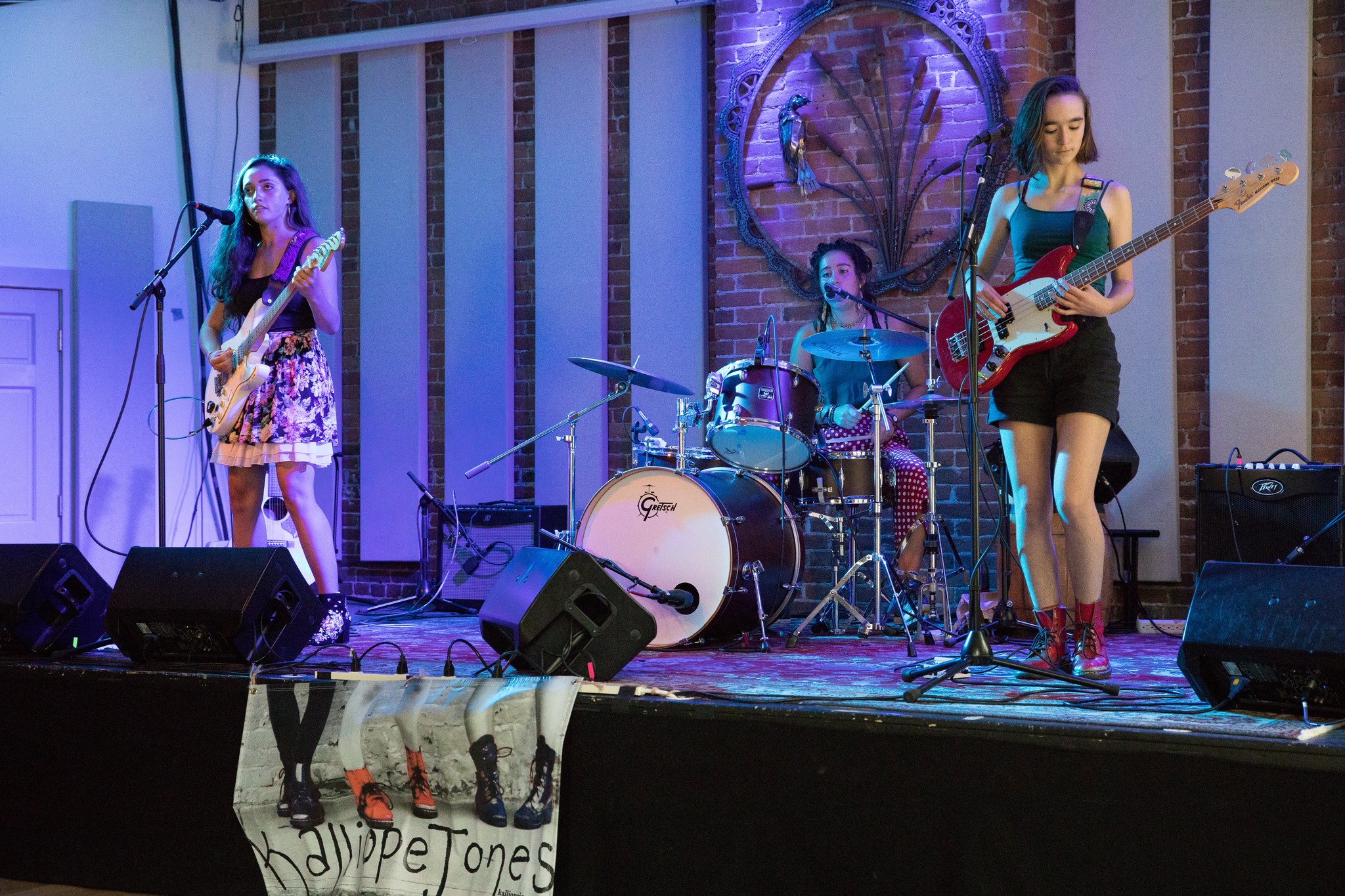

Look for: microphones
[189,201,235,225]
[656,589,694,609]
[463,542,495,574]
[764,322,772,359]
[967,116,1013,148]
[636,408,659,437]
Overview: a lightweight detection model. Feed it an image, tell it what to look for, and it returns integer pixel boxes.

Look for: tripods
[784,355,935,649]
[902,145,1122,704]
[362,471,481,614]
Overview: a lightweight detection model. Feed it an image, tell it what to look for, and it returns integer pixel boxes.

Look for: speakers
[104,547,326,670]
[435,504,542,602]
[478,546,658,681]
[1175,562,1345,720]
[1195,462,1345,565]
[0,542,117,652]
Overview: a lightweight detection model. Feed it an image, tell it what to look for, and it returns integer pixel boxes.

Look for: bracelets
[886,415,894,426]
[818,403,842,428]
[964,275,985,285]
[208,350,214,363]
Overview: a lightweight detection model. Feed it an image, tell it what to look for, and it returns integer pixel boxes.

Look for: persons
[954,76,1136,683]
[201,158,352,649]
[787,239,931,626]
[266,679,573,830]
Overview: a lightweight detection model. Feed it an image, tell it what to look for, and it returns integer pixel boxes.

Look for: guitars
[933,147,1300,398]
[204,225,346,437]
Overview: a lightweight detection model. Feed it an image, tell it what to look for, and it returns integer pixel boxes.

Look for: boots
[289,765,321,825]
[277,768,293,815]
[515,736,557,828]
[344,763,394,827]
[1060,597,1112,679]
[1017,600,1071,679]
[406,747,437,817]
[470,735,523,830]
[899,571,933,625]
[308,592,351,646]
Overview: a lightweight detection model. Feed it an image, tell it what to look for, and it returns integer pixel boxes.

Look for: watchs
[886,412,898,424]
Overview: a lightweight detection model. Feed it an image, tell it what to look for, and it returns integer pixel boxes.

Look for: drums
[572,466,807,653]
[631,443,681,473]
[789,450,897,509]
[682,445,733,472]
[705,357,824,475]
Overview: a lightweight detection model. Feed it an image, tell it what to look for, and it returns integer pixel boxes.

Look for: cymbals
[882,393,986,410]
[800,328,928,362]
[566,356,696,397]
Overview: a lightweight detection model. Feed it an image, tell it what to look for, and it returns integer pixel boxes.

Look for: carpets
[81,615,1345,741]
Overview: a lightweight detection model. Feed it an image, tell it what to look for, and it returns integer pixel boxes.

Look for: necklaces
[829,305,869,331]
